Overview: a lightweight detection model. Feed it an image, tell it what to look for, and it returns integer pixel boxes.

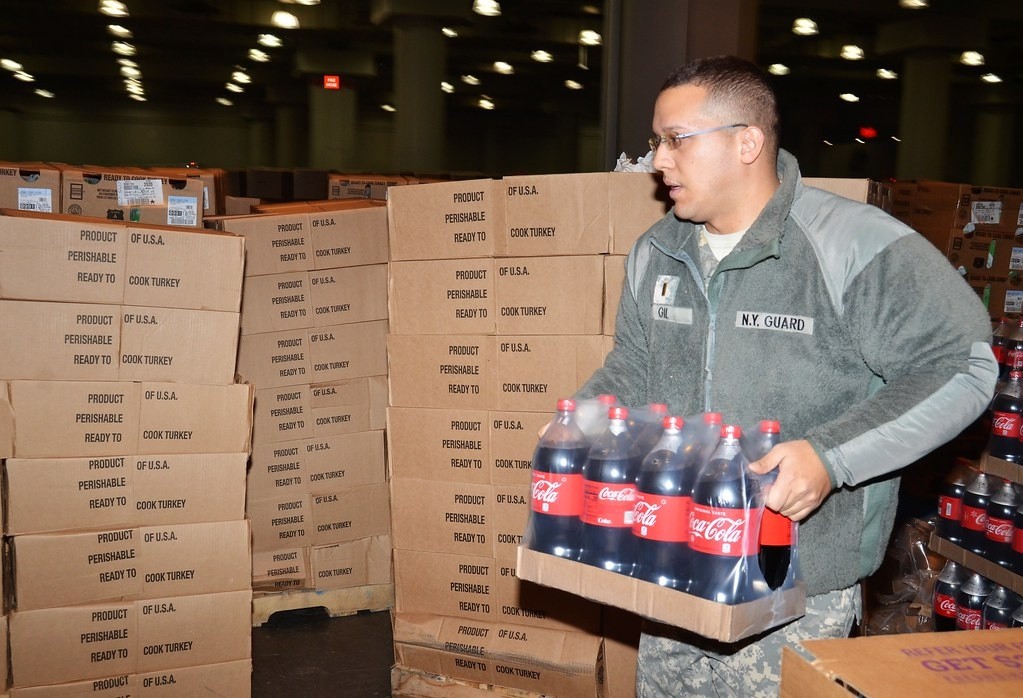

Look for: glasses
[649,123,749,154]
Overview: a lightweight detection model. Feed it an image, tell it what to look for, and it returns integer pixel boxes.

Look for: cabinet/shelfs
[927,445,1022,631]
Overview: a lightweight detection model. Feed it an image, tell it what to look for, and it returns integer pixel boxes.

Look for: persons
[537,55,999,698]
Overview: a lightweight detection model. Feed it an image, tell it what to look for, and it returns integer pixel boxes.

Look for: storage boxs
[1,160,1023,698]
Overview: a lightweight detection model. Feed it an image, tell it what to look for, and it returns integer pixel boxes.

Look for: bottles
[528,393,798,605]
[929,315,1023,632]
